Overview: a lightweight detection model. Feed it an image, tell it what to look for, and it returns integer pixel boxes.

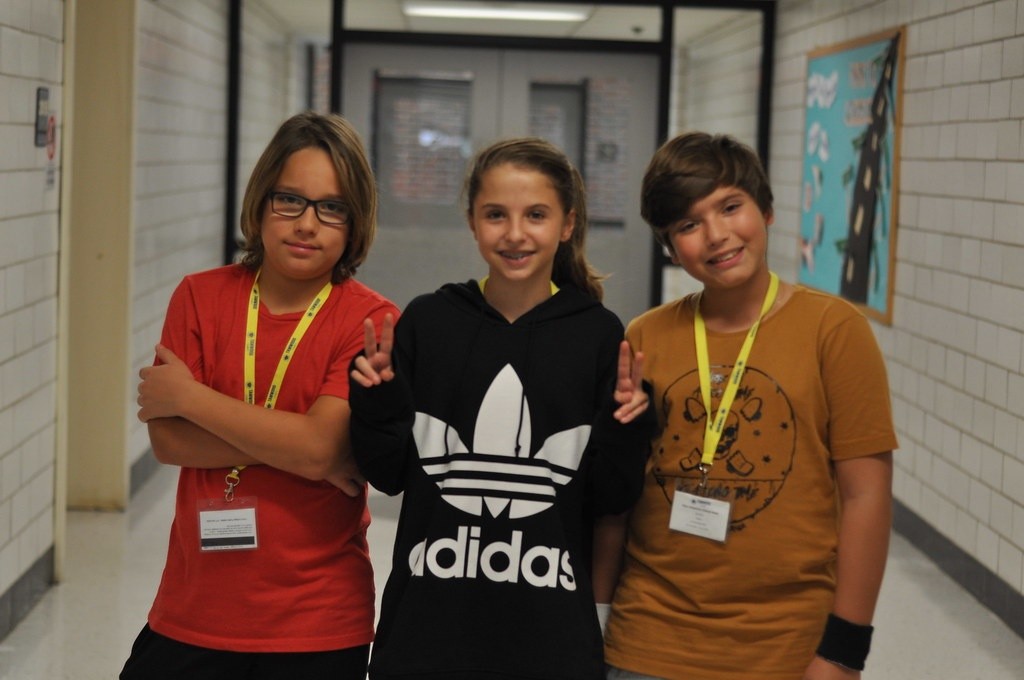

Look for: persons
[593,133,901,680]
[120,110,400,680]
[344,137,655,680]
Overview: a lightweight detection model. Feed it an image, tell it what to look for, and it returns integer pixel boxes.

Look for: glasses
[266,190,352,225]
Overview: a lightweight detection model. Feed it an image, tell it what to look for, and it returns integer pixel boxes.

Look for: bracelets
[815,613,874,672]
[595,603,611,636]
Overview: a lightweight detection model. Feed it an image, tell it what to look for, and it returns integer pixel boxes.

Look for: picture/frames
[798,24,906,327]
